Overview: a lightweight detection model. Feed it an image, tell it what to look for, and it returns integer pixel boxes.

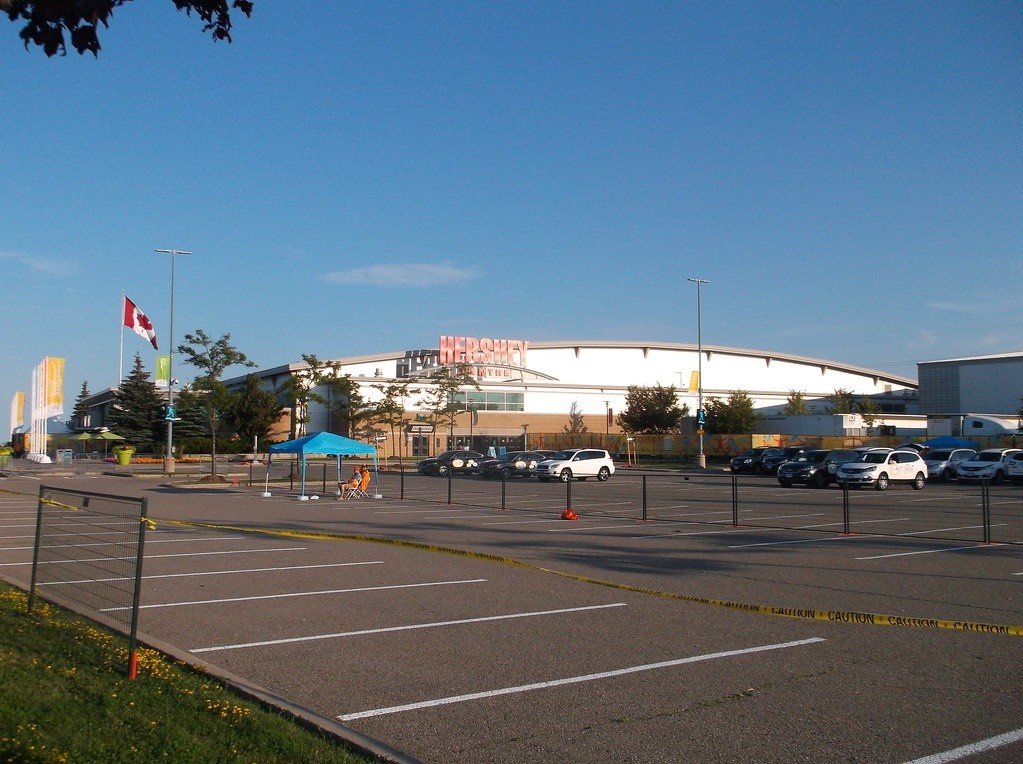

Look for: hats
[354,466,361,471]
[362,463,368,468]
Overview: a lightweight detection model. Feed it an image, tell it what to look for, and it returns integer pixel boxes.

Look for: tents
[921,436,977,448]
[265,431,378,496]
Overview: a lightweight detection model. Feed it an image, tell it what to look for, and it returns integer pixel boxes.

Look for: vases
[0,456,10,467]
[117,450,133,465]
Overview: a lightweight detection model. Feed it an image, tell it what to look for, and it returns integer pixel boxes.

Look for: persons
[338,464,370,500]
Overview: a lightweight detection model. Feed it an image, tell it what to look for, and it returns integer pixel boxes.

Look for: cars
[418,449,483,477]
[922,448,978,482]
[836,450,929,491]
[478,450,546,479]
[956,448,1023,486]
[533,450,565,459]
[854,447,894,456]
[729,445,788,477]
[784,445,821,461]
[777,449,862,488]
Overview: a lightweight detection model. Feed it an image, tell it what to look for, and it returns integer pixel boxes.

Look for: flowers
[0,446,14,456]
[113,444,137,455]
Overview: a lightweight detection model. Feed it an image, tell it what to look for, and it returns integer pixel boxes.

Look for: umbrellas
[67,431,91,453]
[92,431,126,459]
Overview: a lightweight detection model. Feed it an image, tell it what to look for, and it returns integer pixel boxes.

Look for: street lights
[521,424,530,451]
[155,249,192,474]
[688,278,711,468]
[604,401,611,434]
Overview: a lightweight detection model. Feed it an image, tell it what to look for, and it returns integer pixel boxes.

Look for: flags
[124,295,159,350]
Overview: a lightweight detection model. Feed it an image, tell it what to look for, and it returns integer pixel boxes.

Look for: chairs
[344,478,371,501]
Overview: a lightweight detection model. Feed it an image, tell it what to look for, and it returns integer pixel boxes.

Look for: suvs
[534,448,616,482]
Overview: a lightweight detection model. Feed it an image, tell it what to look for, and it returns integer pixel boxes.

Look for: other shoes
[336,497,346,501]
